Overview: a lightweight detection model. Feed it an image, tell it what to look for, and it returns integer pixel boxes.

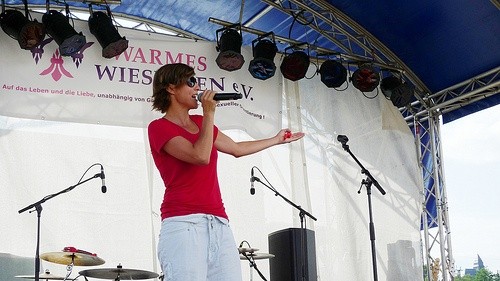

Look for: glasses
[187,76,197,88]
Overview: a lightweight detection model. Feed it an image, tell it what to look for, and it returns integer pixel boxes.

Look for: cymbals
[77,268,159,280]
[39,251,105,267]
[240,253,276,260]
[237,248,260,254]
[14,274,76,281]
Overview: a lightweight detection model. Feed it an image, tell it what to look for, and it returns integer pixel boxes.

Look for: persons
[148,63,305,281]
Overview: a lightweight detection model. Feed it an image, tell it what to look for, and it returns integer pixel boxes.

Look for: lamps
[215,22,413,108]
[0,0,129,59]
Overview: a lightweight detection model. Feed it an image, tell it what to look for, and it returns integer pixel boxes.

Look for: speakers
[268,227,317,281]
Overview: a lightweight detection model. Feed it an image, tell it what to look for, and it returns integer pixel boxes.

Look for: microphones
[249,168,255,195]
[197,92,243,103]
[101,165,106,193]
